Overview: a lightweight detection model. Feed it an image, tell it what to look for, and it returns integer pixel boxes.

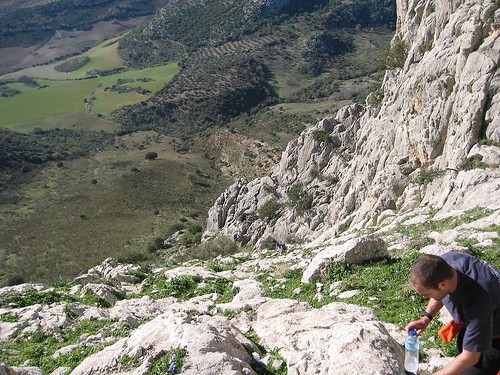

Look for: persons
[405,250,500,375]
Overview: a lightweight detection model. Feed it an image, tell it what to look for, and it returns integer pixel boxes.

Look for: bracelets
[420,311,433,320]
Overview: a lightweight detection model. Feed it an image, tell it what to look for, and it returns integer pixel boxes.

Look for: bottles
[405,329,421,373]
